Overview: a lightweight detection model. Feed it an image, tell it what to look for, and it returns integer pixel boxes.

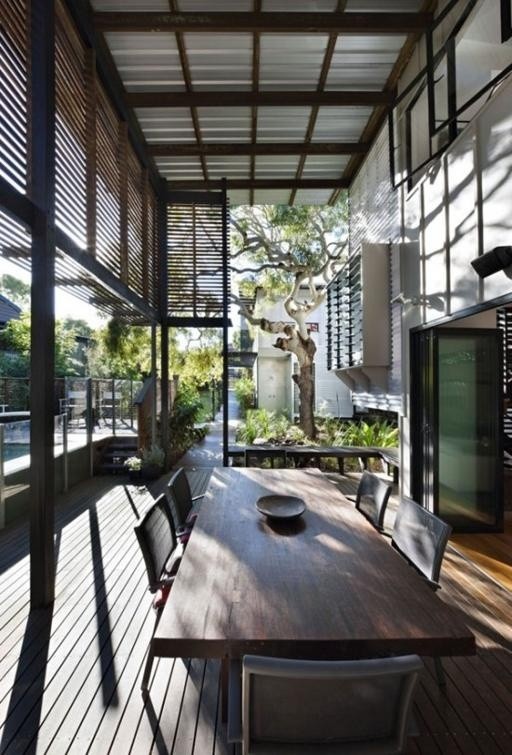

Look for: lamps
[470,245,512,279]
[389,292,417,306]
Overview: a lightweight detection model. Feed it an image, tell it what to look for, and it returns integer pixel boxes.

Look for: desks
[149,464,477,724]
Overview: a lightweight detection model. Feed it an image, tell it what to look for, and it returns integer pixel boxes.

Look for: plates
[254,492,307,521]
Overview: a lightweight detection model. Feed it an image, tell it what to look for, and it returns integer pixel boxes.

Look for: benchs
[224,441,398,473]
